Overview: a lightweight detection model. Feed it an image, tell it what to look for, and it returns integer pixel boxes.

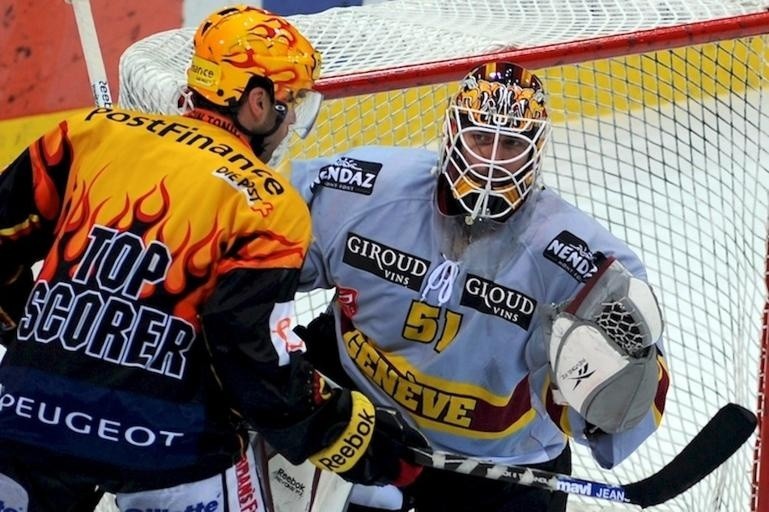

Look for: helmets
[434,60,552,221]
[187,5,323,140]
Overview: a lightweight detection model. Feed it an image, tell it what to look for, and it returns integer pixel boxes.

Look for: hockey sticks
[400,403,757,507]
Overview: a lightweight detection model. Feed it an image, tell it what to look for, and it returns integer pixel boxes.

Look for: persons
[0,7,434,512]
[260,60,670,512]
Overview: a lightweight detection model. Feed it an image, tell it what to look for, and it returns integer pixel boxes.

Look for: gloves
[308,388,434,487]
